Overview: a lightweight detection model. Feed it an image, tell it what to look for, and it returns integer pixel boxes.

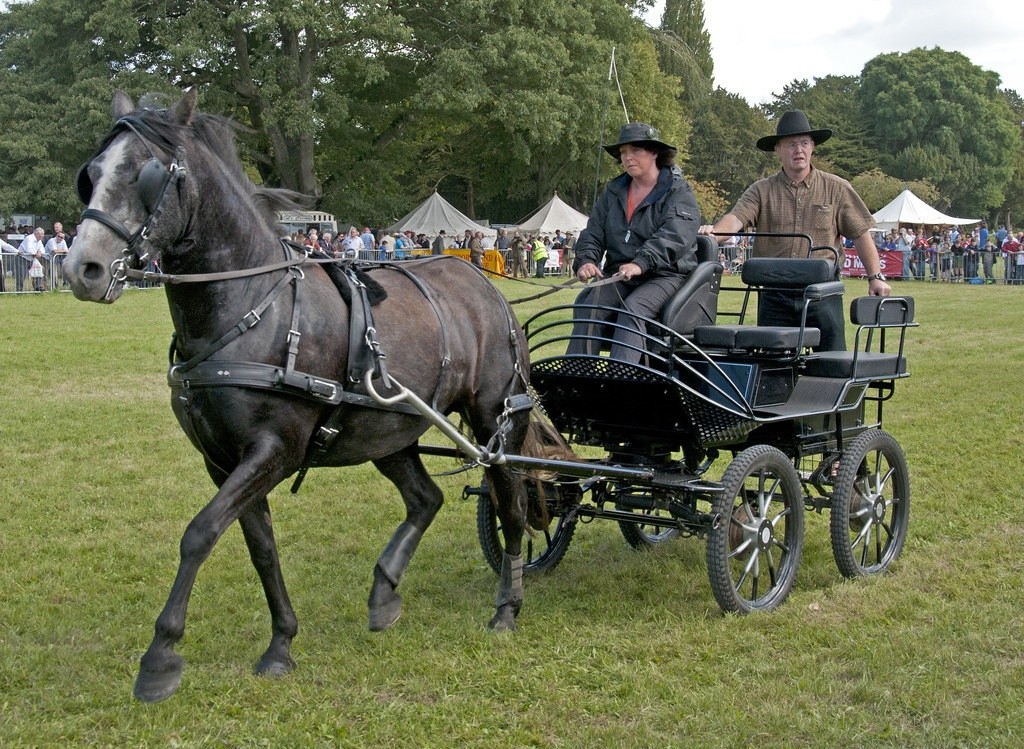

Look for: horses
[60,80,576,703]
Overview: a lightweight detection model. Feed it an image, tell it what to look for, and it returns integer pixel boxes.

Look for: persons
[0,222,85,293]
[698,111,890,351]
[719,233,755,274]
[561,124,701,378]
[290,227,577,279]
[880,223,1024,284]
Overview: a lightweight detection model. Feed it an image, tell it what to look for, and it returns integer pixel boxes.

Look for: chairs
[693,257,845,349]
[572,233,724,336]
[805,294,914,378]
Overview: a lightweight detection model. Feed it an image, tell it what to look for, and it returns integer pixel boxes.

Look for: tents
[871,185,982,237]
[500,191,590,242]
[379,188,498,250]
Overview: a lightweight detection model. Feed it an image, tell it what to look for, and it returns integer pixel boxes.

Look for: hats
[564,231,573,236]
[439,230,446,234]
[602,123,677,161]
[398,233,406,238]
[756,109,832,152]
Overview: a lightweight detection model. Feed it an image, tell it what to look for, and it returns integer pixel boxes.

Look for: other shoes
[987,280,992,284]
[930,274,933,277]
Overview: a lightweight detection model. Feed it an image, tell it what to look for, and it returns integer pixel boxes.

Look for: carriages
[58,79,913,700]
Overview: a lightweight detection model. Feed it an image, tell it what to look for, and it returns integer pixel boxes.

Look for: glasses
[351,231,356,233]
[339,235,344,237]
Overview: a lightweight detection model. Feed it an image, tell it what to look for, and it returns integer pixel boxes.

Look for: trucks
[275,210,338,241]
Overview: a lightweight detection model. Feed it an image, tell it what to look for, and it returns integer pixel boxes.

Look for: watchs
[868,273,886,282]
